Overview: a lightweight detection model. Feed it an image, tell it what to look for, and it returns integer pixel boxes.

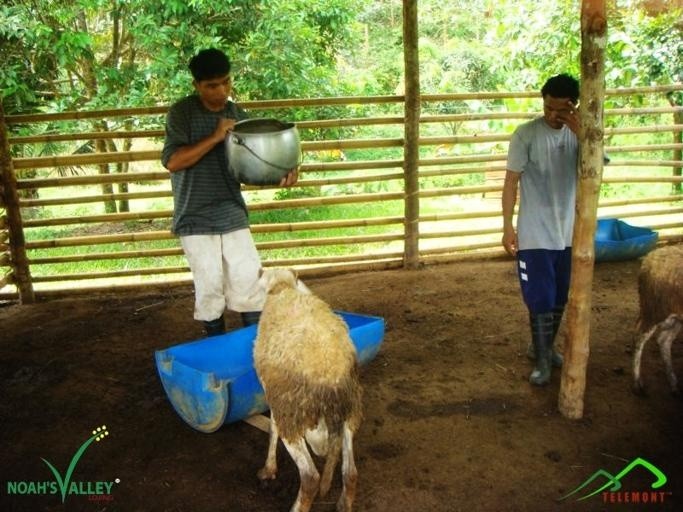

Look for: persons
[499,73,583,385]
[161,48,299,336]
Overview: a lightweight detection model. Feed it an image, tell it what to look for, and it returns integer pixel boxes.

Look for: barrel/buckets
[225,118,303,186]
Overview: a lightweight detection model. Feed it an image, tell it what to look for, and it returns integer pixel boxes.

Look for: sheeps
[633,242,683,398]
[251,268,364,512]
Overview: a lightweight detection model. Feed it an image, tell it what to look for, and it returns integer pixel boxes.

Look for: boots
[242,312,261,326]
[204,313,225,336]
[528,308,564,386]
